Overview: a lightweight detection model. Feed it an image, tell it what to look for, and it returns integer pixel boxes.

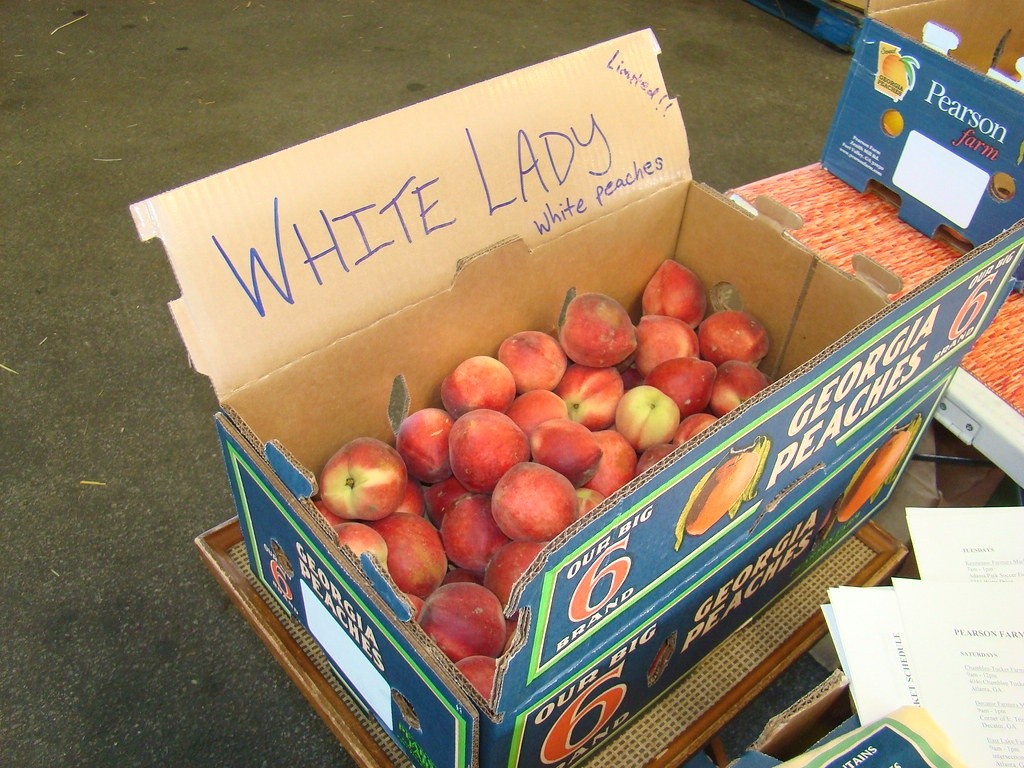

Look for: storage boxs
[128,29,1023,767]
[819,0,1024,297]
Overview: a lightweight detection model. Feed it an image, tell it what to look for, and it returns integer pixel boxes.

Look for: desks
[723,162,1024,507]
[192,516,907,766]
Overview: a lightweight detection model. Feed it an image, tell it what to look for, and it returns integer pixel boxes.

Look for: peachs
[317,257,771,704]
